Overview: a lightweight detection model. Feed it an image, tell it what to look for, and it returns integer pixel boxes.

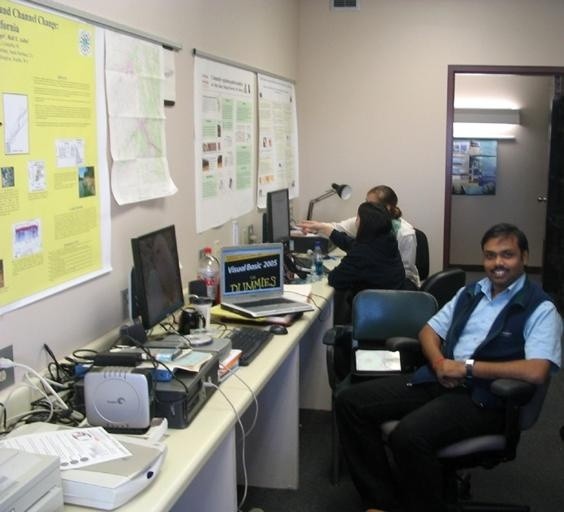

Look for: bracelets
[432,357,444,368]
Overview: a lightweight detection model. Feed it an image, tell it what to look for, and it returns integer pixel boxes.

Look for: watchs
[464,359,475,379]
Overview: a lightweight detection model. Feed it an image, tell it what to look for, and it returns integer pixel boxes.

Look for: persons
[334,222,563,512]
[301,185,421,289]
[295,201,405,349]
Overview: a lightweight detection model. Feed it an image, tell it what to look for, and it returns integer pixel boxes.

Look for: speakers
[189,280,206,304]
[128,325,147,344]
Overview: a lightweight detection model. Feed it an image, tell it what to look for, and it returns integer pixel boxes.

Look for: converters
[94,351,142,367]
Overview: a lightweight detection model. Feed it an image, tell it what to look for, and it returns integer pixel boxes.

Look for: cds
[184,334,211,345]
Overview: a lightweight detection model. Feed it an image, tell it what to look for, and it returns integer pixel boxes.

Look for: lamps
[307,183,352,220]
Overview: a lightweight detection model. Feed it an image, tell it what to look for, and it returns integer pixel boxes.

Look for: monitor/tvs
[267,188,289,254]
[131,224,184,342]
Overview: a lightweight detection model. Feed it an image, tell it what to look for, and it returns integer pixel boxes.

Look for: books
[135,351,213,373]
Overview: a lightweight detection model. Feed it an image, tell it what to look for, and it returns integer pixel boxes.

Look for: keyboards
[228,327,274,366]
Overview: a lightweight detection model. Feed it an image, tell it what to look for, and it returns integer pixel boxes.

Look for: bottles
[248,224,255,243]
[312,241,323,280]
[197,246,221,304]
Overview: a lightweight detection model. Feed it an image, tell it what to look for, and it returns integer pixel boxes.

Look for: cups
[178,307,206,335]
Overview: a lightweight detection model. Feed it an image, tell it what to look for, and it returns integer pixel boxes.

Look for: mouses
[270,324,288,334]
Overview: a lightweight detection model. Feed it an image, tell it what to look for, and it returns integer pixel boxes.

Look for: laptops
[220,242,314,318]
